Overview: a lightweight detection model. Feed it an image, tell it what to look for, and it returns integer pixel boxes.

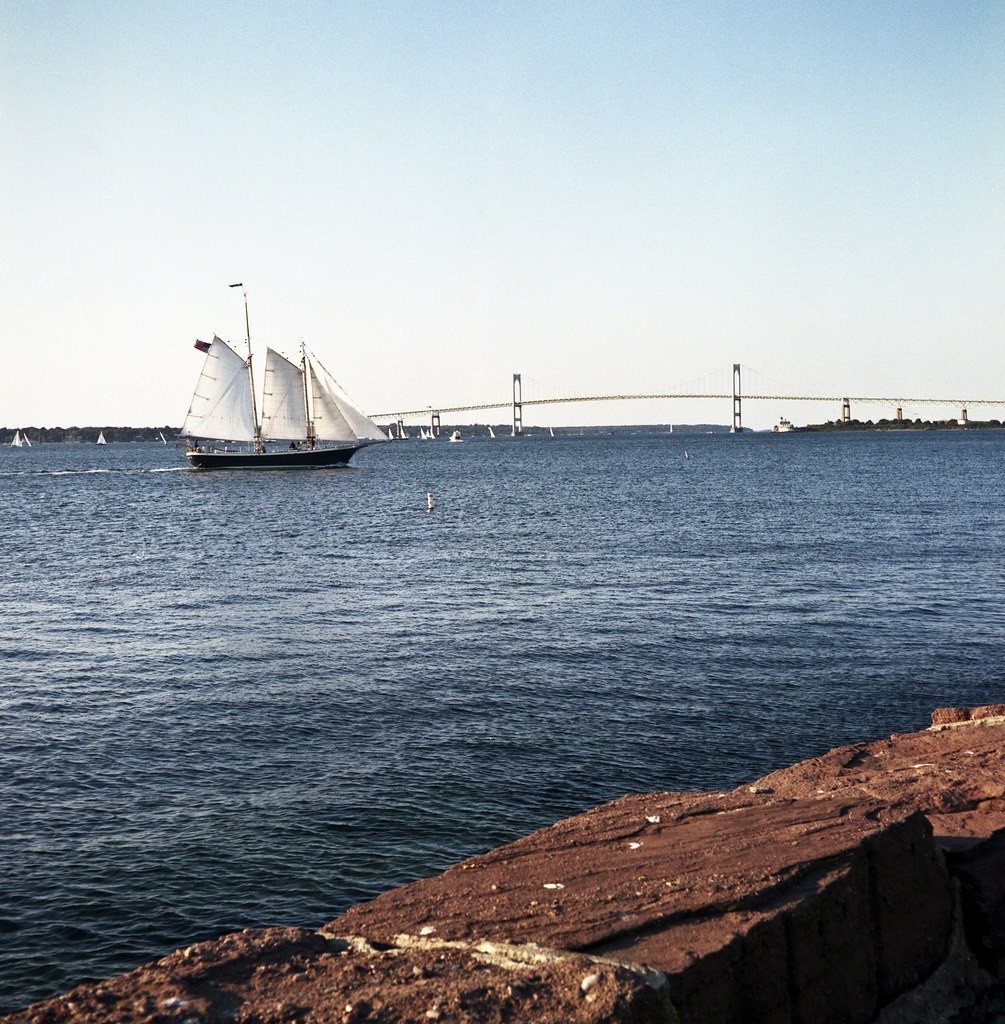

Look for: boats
[771,417,797,433]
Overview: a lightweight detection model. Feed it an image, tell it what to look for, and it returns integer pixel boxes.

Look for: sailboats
[488,427,495,438]
[394,419,409,440]
[449,433,464,443]
[388,427,393,443]
[420,427,435,441]
[10,431,32,450]
[175,282,410,472]
[96,431,107,445]
[669,424,673,433]
[160,432,168,446]
[550,426,554,437]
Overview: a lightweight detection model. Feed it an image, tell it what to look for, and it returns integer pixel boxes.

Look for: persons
[194,439,201,452]
[186,437,192,447]
[289,441,295,450]
[296,441,301,450]
